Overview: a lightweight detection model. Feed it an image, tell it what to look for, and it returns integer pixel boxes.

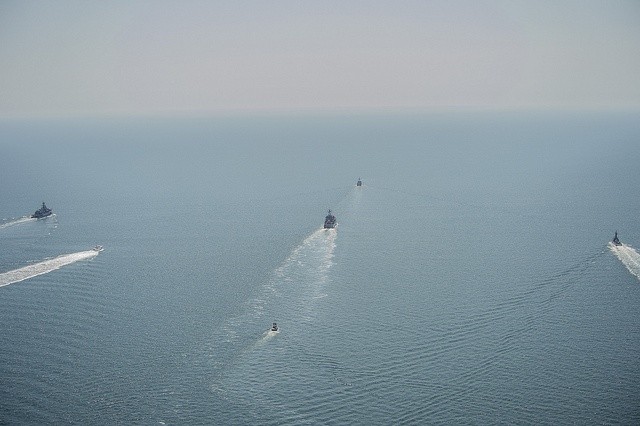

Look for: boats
[612,231,622,247]
[357,177,362,186]
[271,323,279,331]
[93,243,104,251]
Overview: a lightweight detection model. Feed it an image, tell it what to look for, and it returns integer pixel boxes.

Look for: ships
[31,202,52,219]
[324,209,338,228]
